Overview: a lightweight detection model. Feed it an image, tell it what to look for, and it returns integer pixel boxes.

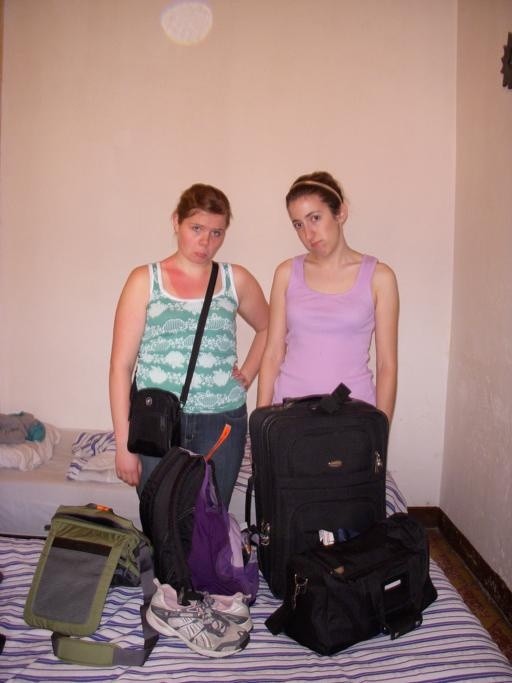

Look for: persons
[256,171,399,431]
[108,184,269,513]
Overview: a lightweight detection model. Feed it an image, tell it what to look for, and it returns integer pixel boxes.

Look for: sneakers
[146,578,253,658]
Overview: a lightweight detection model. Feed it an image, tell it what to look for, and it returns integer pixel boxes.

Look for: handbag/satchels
[23,503,154,636]
[187,510,259,606]
[127,388,181,457]
[285,513,437,656]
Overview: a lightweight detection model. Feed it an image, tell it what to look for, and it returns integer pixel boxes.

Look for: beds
[1,536,512,683]
[1,414,407,539]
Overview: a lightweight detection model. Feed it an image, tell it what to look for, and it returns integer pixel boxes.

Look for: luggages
[245,383,389,599]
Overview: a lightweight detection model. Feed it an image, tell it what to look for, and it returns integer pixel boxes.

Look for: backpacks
[140,446,251,605]
